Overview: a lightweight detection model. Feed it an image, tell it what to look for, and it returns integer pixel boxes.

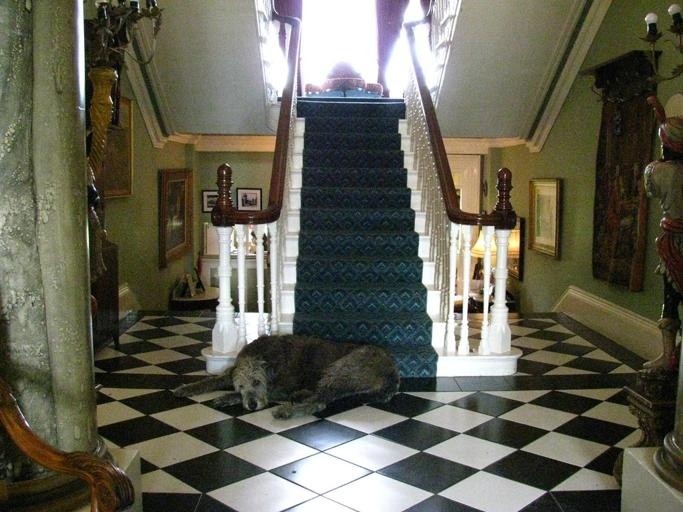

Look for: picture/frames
[102,94,136,200]
[156,165,264,270]
[502,175,562,282]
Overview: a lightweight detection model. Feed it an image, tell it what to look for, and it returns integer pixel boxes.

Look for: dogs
[173,334,402,419]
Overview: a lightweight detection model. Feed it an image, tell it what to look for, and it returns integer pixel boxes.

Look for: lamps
[638,0,681,85]
[472,229,498,292]
